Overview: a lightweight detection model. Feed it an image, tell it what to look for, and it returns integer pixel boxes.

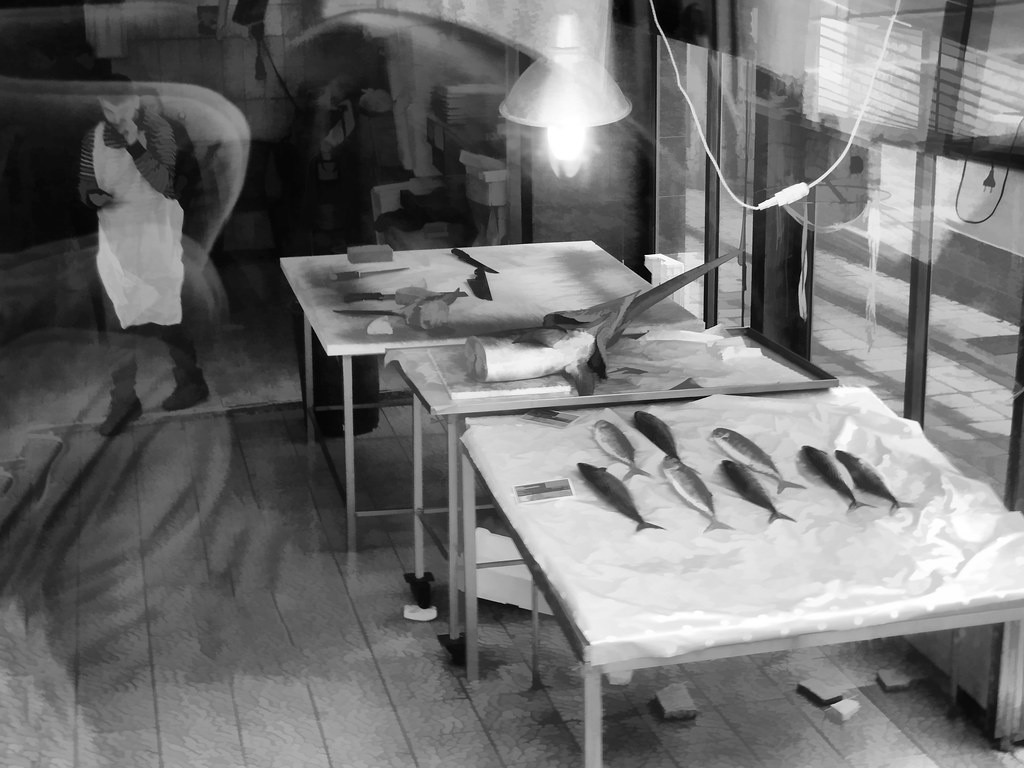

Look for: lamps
[498,12,632,179]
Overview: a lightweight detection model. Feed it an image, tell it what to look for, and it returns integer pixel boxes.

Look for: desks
[383,326,842,669]
[278,238,708,558]
[458,388,1024,768]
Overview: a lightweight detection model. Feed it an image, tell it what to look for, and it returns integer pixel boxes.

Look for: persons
[76,74,211,437]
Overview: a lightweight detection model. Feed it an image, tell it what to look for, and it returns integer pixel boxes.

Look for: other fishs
[835,449,920,515]
[463,246,745,396]
[800,441,876,517]
[720,460,794,523]
[590,416,651,484]
[580,461,661,533]
[712,428,805,492]
[632,407,686,468]
[658,451,734,535]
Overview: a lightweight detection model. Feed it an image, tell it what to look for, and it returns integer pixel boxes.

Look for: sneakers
[161,366,211,412]
[96,384,142,437]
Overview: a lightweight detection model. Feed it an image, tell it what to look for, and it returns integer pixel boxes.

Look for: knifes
[329,267,410,281]
[451,247,498,273]
[344,292,400,302]
[467,267,493,301]
